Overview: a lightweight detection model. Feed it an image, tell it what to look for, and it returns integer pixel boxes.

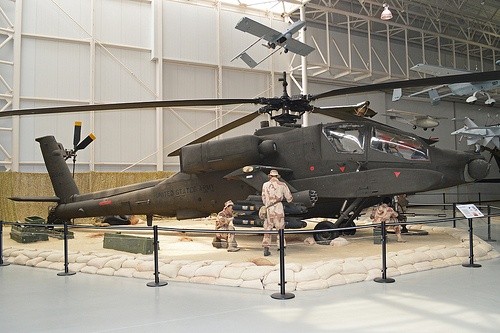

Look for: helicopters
[0,71,499,250]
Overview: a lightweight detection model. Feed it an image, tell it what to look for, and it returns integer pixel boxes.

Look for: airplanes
[230,16,315,69]
[380,108,450,132]
[405,62,500,106]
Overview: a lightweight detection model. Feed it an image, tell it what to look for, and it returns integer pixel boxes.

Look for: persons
[392,194,409,233]
[332,129,347,152]
[370,197,407,243]
[262,169,293,256]
[212,200,240,252]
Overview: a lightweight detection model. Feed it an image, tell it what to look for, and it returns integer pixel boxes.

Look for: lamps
[380,4,393,20]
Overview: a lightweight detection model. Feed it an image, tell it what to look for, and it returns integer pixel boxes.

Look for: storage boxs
[103,233,153,255]
[9,216,74,243]
